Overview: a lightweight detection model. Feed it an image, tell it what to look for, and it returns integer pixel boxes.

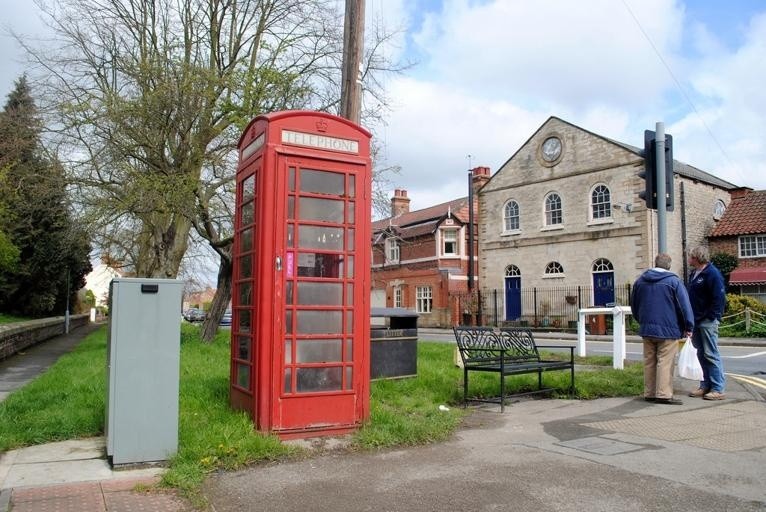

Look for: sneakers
[704,391,725,400]
[645,396,684,405]
[689,389,710,397]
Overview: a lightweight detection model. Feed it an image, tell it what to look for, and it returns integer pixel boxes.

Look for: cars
[183,308,232,326]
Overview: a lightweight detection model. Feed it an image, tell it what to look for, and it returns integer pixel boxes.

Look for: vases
[463,313,472,326]
[535,320,540,326]
[553,320,560,327]
[568,320,577,327]
[520,321,527,327]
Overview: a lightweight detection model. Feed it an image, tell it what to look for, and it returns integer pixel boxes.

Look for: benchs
[453,327,576,413]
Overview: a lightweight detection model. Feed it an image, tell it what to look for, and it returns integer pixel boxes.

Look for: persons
[688,244,728,401]
[630,254,695,403]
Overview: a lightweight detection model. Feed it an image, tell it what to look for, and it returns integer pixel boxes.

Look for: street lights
[66,264,70,334]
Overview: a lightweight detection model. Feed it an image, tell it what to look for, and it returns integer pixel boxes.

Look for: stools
[500,320,516,326]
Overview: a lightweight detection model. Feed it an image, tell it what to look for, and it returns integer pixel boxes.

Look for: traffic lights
[638,129,674,210]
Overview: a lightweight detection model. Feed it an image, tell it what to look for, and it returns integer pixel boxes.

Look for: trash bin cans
[370,307,419,382]
